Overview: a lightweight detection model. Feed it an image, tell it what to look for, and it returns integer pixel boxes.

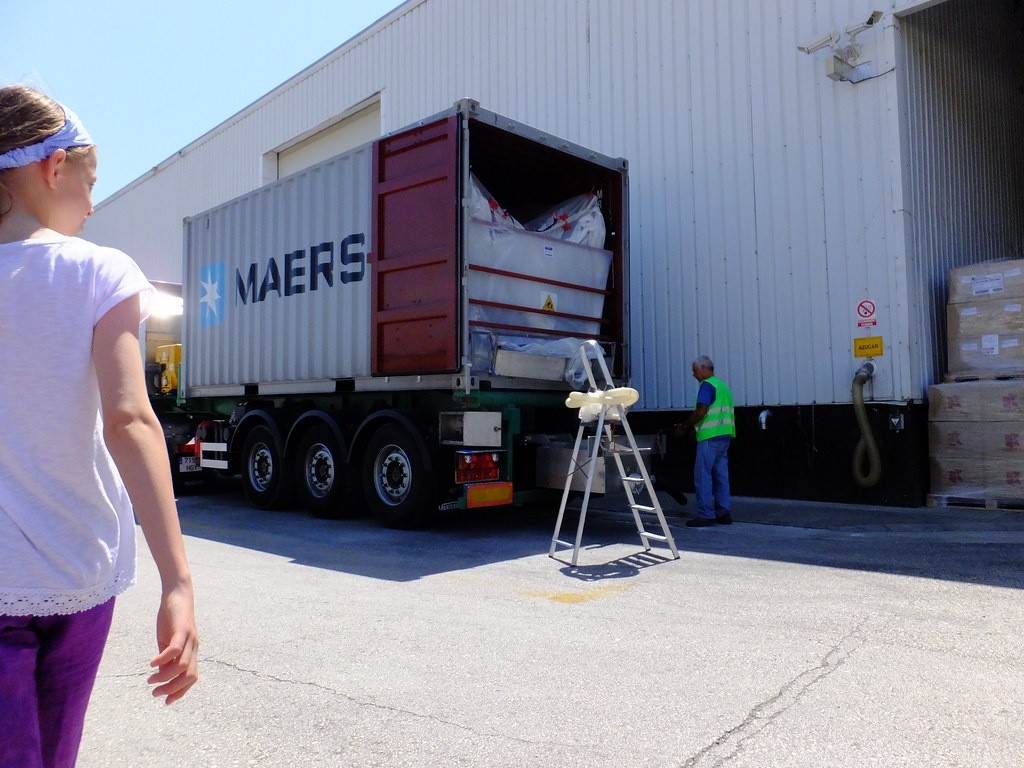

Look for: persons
[0,82,202,768]
[682,357,737,526]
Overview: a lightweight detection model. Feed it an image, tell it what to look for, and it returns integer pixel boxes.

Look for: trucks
[137,95,638,535]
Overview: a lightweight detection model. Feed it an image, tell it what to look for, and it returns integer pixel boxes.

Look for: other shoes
[685,512,733,527]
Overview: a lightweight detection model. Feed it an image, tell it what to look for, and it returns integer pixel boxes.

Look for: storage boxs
[468,217,614,347]
[928,379,1024,505]
[946,259,1024,380]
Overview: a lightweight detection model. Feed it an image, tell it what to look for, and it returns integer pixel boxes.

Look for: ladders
[548,339,680,567]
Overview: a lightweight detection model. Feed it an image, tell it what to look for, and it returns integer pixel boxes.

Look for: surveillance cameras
[796,10,884,61]
